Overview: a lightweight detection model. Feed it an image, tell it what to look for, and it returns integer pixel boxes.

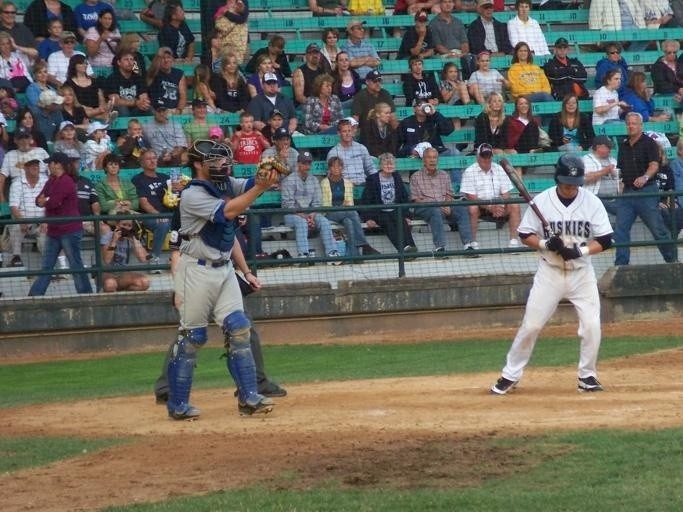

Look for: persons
[491,153,614,397]
[1,0,683,290]
[166,139,277,418]
[154,144,287,404]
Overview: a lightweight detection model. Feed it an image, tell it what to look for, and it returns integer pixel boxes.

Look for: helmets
[553,153,587,188]
[189,140,236,183]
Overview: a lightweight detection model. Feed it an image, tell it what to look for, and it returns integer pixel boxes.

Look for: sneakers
[296,236,521,268]
[147,254,163,276]
[238,392,276,415]
[576,373,604,393]
[10,256,24,266]
[155,375,170,403]
[167,405,204,419]
[255,381,287,398]
[490,376,518,395]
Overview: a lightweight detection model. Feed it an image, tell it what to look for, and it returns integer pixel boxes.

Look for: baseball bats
[500,158,554,237]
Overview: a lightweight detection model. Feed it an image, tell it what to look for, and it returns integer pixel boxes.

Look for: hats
[367,70,382,79]
[477,141,493,157]
[298,151,313,162]
[306,43,320,52]
[347,20,367,29]
[413,97,428,106]
[593,135,615,147]
[25,30,291,153]
[414,11,429,21]
[556,38,569,47]
[477,1,494,7]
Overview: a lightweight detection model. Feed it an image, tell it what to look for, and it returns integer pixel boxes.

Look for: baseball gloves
[256,153,290,191]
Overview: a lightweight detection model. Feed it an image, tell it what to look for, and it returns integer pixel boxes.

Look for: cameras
[120,227,131,238]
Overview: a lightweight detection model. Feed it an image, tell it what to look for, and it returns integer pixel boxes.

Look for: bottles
[137,136,146,149]
[169,167,181,194]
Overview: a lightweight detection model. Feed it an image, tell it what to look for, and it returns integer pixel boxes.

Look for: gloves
[560,245,583,263]
[539,235,562,252]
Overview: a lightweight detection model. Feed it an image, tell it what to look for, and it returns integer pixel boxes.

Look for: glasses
[483,6,492,10]
[610,51,619,54]
[369,79,382,82]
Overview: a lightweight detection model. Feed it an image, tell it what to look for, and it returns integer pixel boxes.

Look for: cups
[663,105,672,116]
[611,167,620,180]
[59,255,67,267]
[421,104,431,113]
[308,248,315,265]
